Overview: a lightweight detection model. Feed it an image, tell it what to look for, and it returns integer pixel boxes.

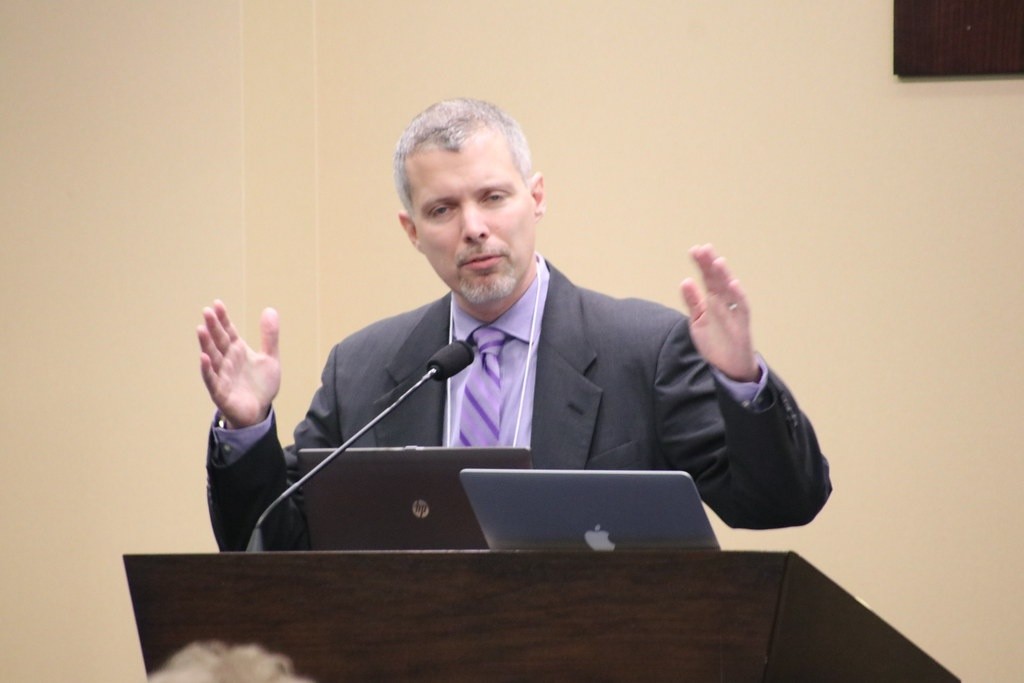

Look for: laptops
[297,448,532,550]
[459,468,719,552]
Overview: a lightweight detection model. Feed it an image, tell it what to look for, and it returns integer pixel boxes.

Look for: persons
[196,97,832,554]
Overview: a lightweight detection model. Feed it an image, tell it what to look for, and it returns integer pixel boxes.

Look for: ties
[453,327,515,448]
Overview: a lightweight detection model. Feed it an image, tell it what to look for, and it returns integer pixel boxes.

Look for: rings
[727,303,736,313]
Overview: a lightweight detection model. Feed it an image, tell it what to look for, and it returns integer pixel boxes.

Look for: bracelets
[219,414,227,430]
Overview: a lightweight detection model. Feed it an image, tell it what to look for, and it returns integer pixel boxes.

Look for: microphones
[245,341,475,551]
